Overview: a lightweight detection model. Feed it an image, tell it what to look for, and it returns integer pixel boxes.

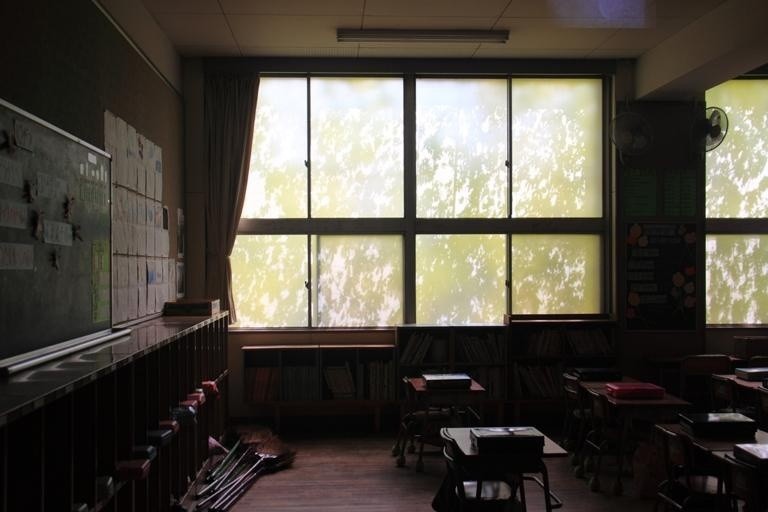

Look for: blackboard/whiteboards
[0,99,132,374]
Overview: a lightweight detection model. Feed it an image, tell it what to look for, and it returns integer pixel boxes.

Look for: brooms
[193,421,299,511]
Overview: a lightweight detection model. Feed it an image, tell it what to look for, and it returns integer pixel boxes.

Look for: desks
[392,352,768,512]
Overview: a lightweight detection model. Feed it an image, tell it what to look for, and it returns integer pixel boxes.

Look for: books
[606,381,665,400]
[759,377,768,389]
[734,365,767,381]
[731,442,768,467]
[678,409,758,434]
[467,423,546,455]
[162,299,223,318]
[422,371,474,391]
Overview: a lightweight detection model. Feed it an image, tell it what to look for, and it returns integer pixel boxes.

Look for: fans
[610,96,654,158]
[690,95,728,152]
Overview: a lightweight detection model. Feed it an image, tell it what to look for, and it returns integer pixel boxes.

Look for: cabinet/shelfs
[0,309,230,512]
[398,325,510,426]
[225,329,399,419]
[510,319,624,401]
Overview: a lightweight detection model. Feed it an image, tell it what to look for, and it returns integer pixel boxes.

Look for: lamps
[336,28,509,43]
